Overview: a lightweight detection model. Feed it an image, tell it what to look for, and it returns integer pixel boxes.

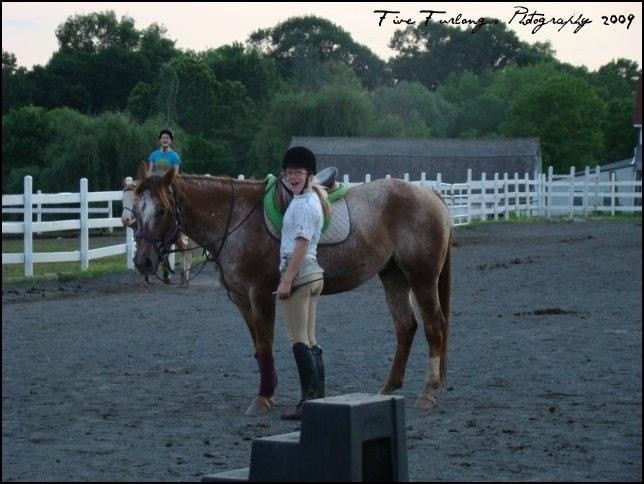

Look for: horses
[122,159,454,419]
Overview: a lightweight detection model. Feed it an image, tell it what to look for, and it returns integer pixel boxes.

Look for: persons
[276,146,332,420]
[148,128,182,181]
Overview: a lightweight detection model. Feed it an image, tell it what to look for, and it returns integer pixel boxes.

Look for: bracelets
[280,276,293,284]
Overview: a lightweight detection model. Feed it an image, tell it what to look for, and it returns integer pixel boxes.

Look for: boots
[281,343,324,420]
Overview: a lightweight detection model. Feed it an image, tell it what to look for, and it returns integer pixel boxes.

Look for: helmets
[282,147,316,176]
[158,129,174,140]
